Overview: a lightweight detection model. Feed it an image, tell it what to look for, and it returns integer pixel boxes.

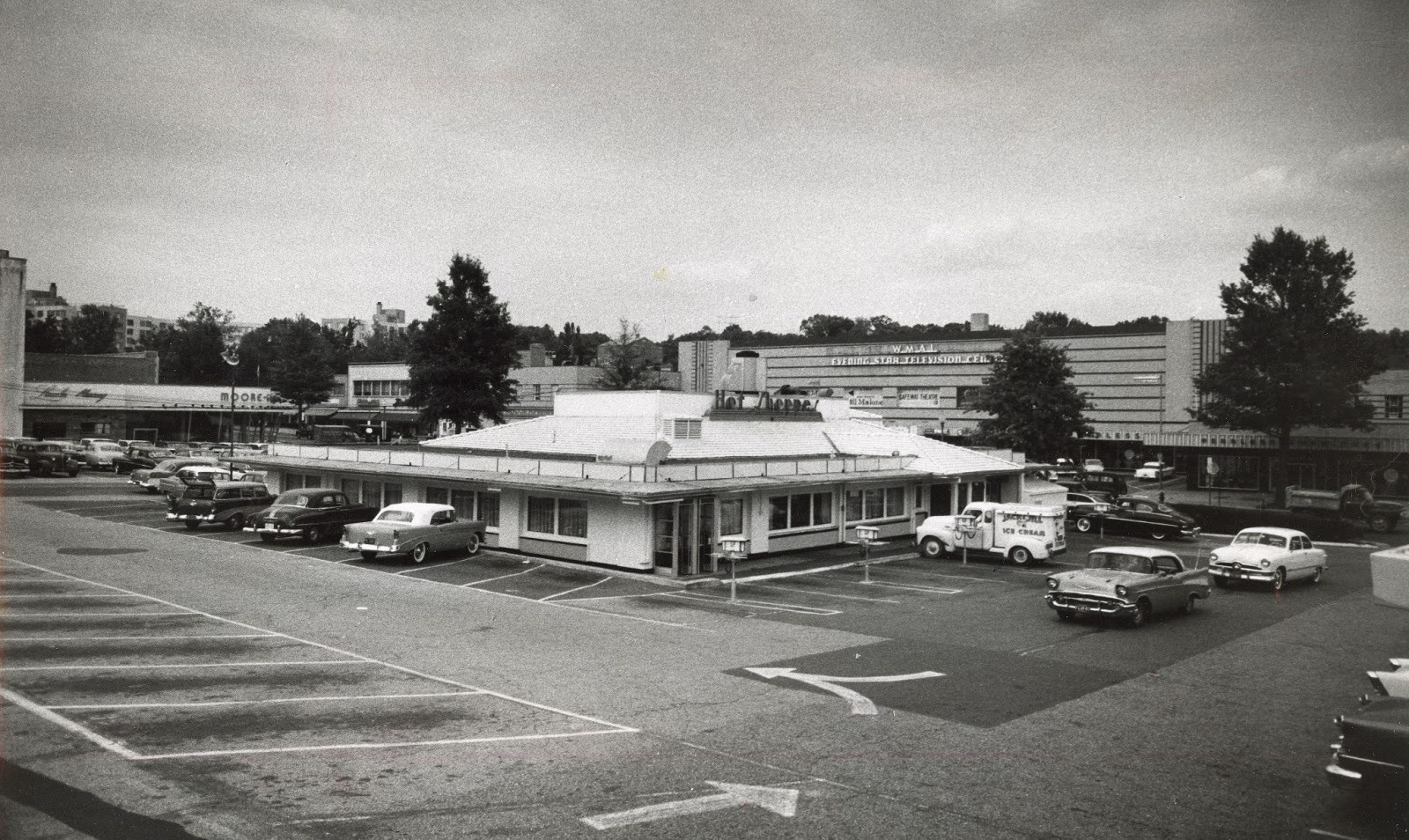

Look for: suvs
[14,442,79,478]
[166,480,282,532]
[0,437,31,479]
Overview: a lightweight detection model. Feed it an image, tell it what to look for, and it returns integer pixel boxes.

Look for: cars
[1067,492,1112,521]
[159,465,256,504]
[1207,526,1330,593]
[338,502,487,566]
[1134,461,1176,482]
[1359,657,1409,705]
[79,439,281,494]
[243,487,379,546]
[1043,545,1211,628]
[1324,692,1409,800]
[1025,458,1128,496]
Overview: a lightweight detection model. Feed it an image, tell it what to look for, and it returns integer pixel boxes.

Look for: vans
[354,425,392,443]
[314,425,357,444]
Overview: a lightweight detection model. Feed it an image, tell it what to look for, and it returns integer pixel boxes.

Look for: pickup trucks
[915,500,1067,567]
[62,441,127,471]
[1284,483,1405,534]
[1067,495,1202,541]
